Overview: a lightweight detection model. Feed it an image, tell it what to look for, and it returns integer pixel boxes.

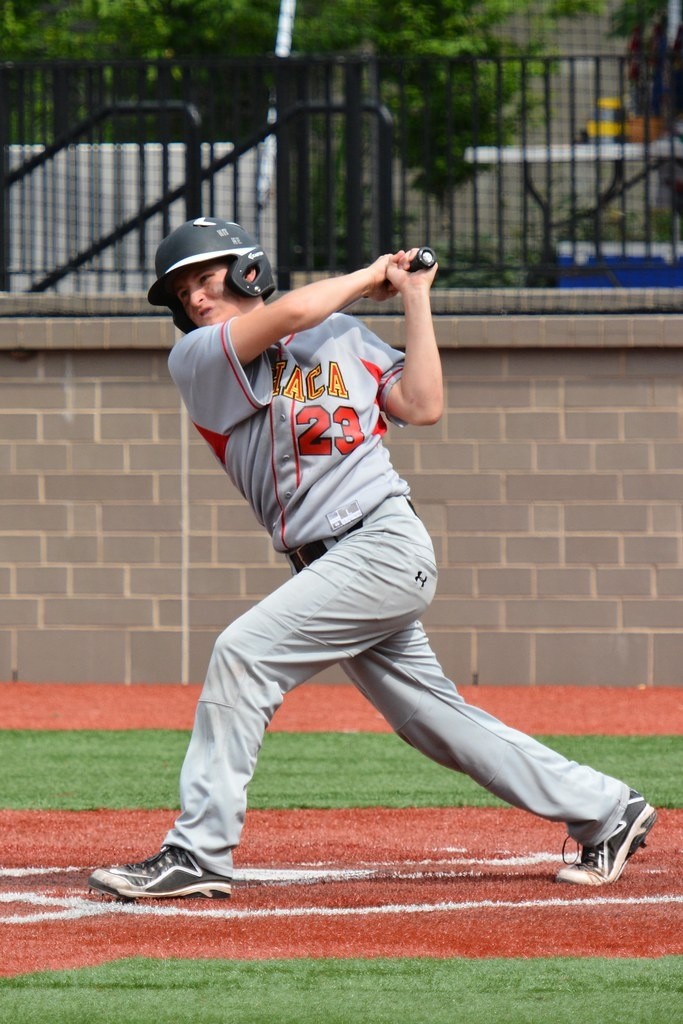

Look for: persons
[88,215,662,900]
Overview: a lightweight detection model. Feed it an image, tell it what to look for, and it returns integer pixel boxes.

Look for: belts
[288,512,363,576]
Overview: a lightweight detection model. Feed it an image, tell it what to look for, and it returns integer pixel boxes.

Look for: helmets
[147,216,276,334]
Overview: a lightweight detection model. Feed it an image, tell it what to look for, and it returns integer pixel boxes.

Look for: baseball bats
[336,246,437,313]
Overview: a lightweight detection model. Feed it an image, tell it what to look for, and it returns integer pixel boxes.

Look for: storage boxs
[558,242,683,288]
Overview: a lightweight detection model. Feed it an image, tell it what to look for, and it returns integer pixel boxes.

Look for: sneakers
[555,787,659,887]
[88,842,234,900]
